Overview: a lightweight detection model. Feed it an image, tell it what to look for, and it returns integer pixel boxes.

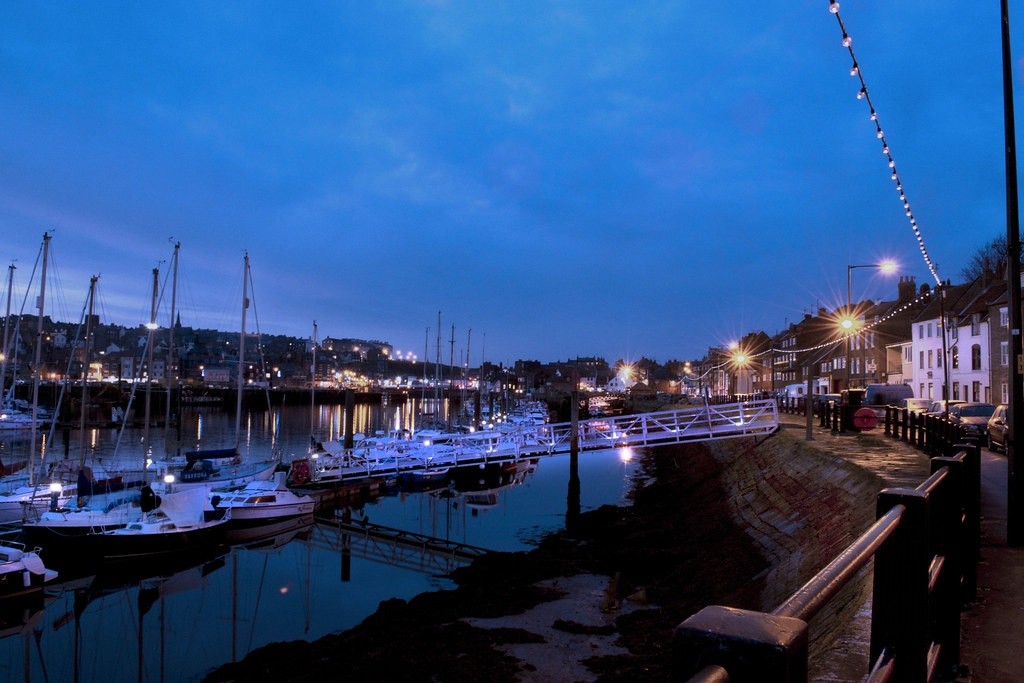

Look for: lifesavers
[292,470,306,484]
[854,407,878,431]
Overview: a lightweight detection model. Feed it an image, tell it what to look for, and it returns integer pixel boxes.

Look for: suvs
[841,389,866,405]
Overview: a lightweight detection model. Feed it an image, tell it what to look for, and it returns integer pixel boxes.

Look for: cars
[799,393,841,409]
[657,391,785,399]
[899,398,1009,456]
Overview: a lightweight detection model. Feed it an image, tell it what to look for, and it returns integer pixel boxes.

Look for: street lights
[842,263,894,387]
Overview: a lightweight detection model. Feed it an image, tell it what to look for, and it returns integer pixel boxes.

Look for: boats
[288,310,549,484]
[0,229,316,599]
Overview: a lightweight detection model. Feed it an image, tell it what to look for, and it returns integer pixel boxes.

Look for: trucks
[781,384,814,408]
[865,384,914,406]
